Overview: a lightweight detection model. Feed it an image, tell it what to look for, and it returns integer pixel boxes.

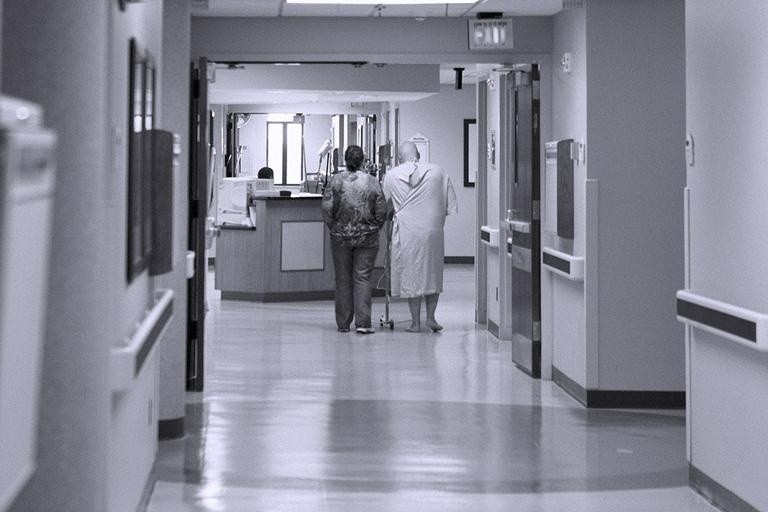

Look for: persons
[381,139,460,335]
[257,167,275,185]
[321,145,388,335]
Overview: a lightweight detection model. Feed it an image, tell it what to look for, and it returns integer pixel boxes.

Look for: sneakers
[337,327,351,332]
[355,324,376,334]
